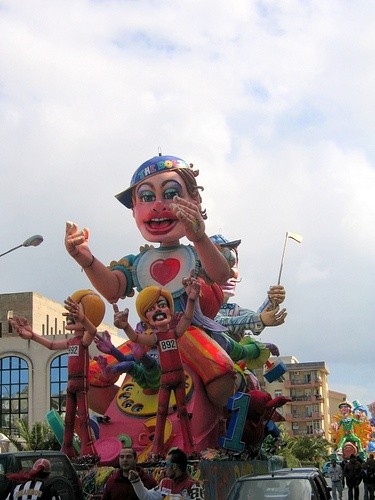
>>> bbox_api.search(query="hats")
[33,459,51,473]
[350,454,355,459]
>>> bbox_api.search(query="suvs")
[0,452,81,500]
[220,466,334,500]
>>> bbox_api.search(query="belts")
[332,480,340,482]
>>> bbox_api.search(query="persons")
[7,290,108,459]
[326,455,375,499]
[331,398,375,454]
[199,234,287,342]
[114,277,208,462]
[92,310,184,391]
[129,448,204,500]
[7,459,61,500]
[65,154,236,409]
[104,447,159,500]
